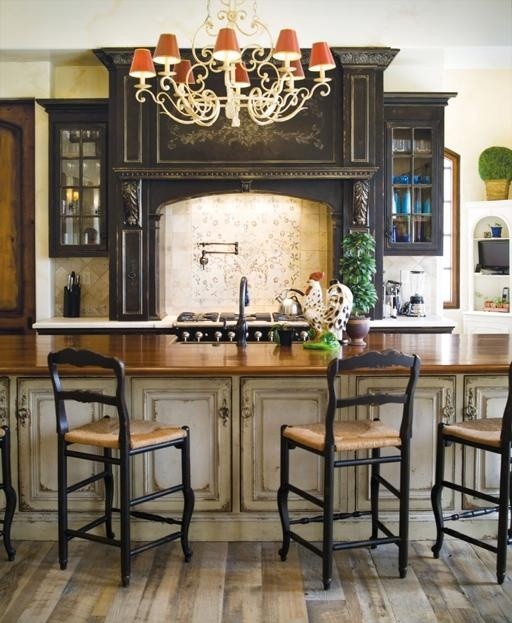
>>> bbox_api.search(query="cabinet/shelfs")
[48,117,111,257]
[384,117,443,257]
[1,374,125,515]
[354,370,512,514]
[129,372,353,523]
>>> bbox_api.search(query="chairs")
[47,347,194,585]
[276,350,421,589]
[431,363,512,585]
[0,424,17,560]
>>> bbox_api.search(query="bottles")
[393,190,430,243]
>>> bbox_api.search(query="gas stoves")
[175,310,311,345]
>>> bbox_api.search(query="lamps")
[129,1,340,128]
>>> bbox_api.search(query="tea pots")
[275,288,305,315]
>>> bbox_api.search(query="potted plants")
[339,232,378,346]
[479,147,512,201]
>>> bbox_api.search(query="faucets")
[235,276,249,346]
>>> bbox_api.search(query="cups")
[63,286,81,318]
[393,175,431,185]
[484,226,502,238]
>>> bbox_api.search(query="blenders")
[409,270,426,317]
